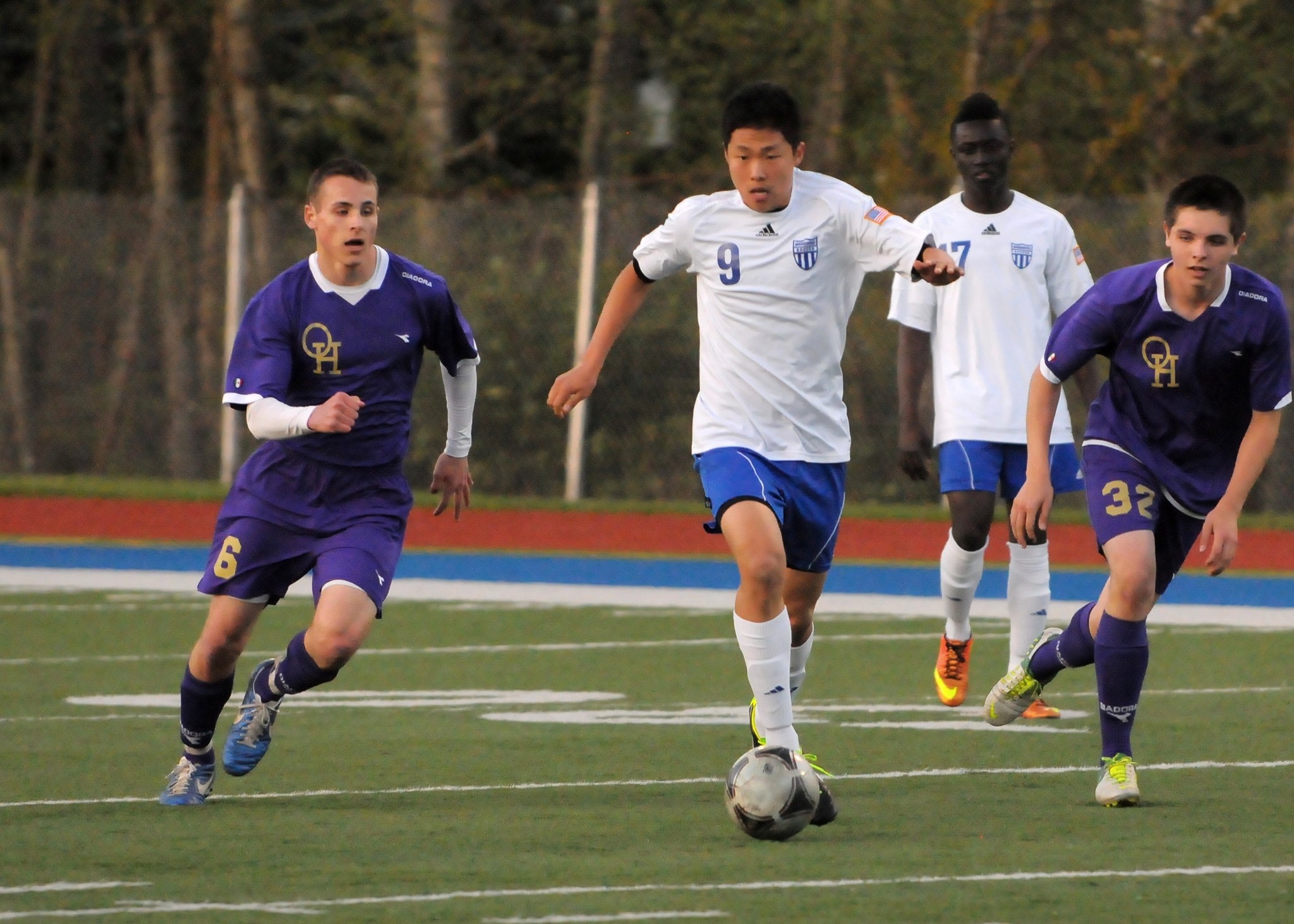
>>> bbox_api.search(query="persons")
[547,79,964,829]
[888,93,1105,718]
[161,157,482,806]
[983,175,1294,805]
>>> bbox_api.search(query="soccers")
[722,744,823,843]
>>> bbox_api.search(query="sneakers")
[934,632,973,706]
[223,657,286,777]
[160,756,216,805]
[1095,753,1141,806]
[983,628,1064,727]
[747,697,837,828]
[1021,700,1061,719]
